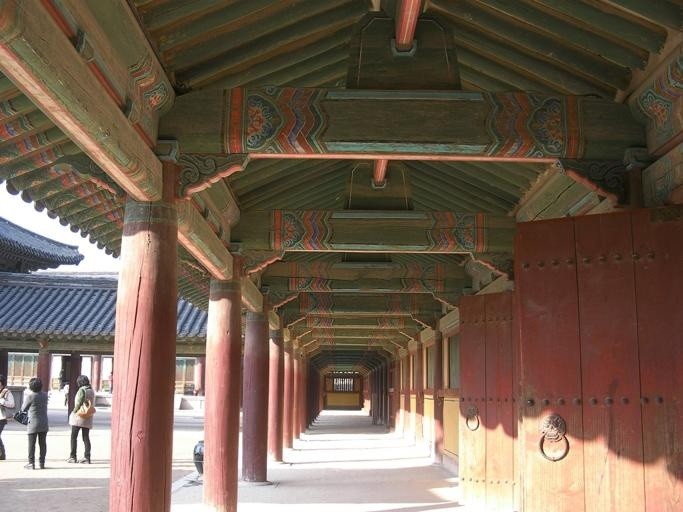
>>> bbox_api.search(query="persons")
[63,375,95,465]
[0,373,15,459]
[19,377,50,469]
[63,382,69,405]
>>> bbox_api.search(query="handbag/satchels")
[77,400,97,419]
[12,410,29,425]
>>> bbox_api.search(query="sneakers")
[64,456,91,465]
[23,463,46,471]
